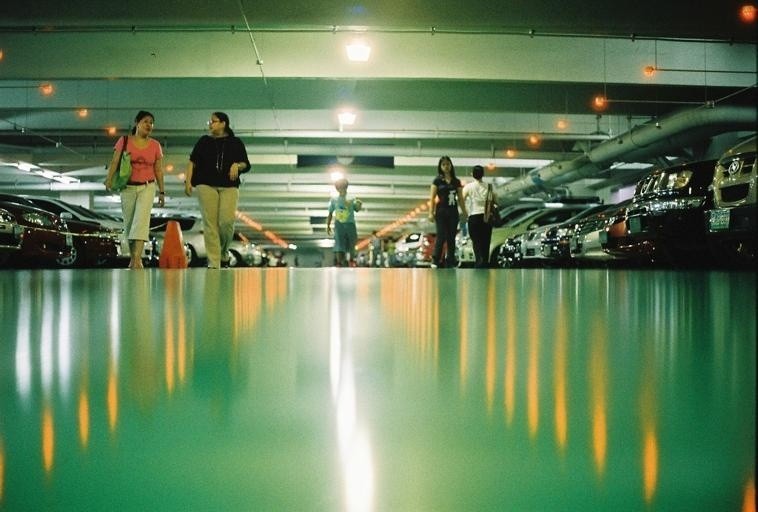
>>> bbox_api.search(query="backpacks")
[105,134,135,193]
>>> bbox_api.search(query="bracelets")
[159,191,165,194]
[237,162,240,169]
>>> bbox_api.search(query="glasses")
[206,118,220,125]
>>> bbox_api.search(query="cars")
[0,194,270,267]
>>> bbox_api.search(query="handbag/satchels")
[184,165,196,189]
[482,183,504,227]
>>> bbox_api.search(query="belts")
[126,178,155,186]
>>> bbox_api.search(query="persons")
[461,164,498,269]
[185,109,251,269]
[102,109,165,269]
[325,178,362,267]
[369,230,381,267]
[384,235,396,267]
[427,155,467,269]
